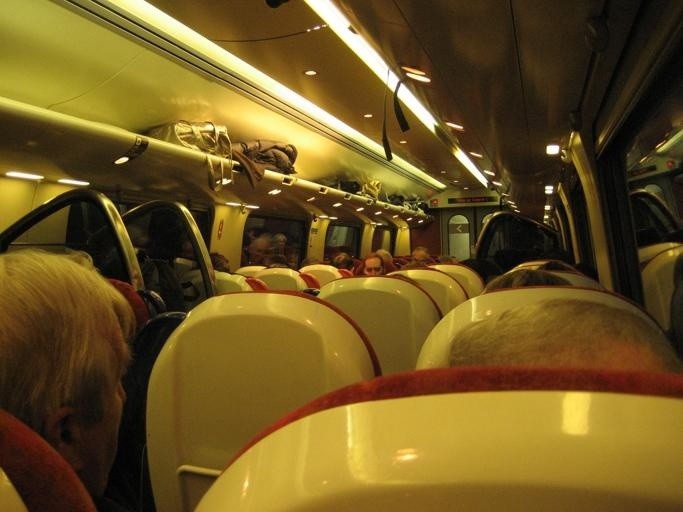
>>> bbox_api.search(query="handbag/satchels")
[144,120,230,158]
[231,139,296,174]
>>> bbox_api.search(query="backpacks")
[318,175,430,213]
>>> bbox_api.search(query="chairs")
[638,242,682,334]
[144,258,683,512]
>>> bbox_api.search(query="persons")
[70,222,354,281]
[201,244,578,296]
[58,249,95,272]
[446,298,682,377]
[0,242,136,512]
